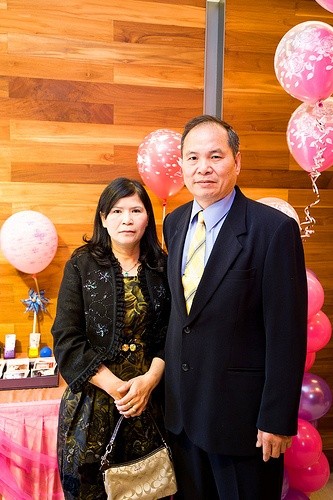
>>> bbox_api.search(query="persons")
[163,115,309,500]
[50,177,172,500]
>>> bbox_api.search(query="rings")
[131,407,137,413]
[127,402,132,409]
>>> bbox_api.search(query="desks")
[0,371,68,500]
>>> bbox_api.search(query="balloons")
[257,197,333,500]
[0,211,58,275]
[273,20,333,172]
[137,128,184,207]
[315,0,333,13]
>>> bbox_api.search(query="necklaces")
[122,263,138,276]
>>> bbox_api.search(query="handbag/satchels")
[99,404,178,500]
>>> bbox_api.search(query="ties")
[182,210,207,317]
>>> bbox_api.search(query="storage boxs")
[0,360,59,390]
